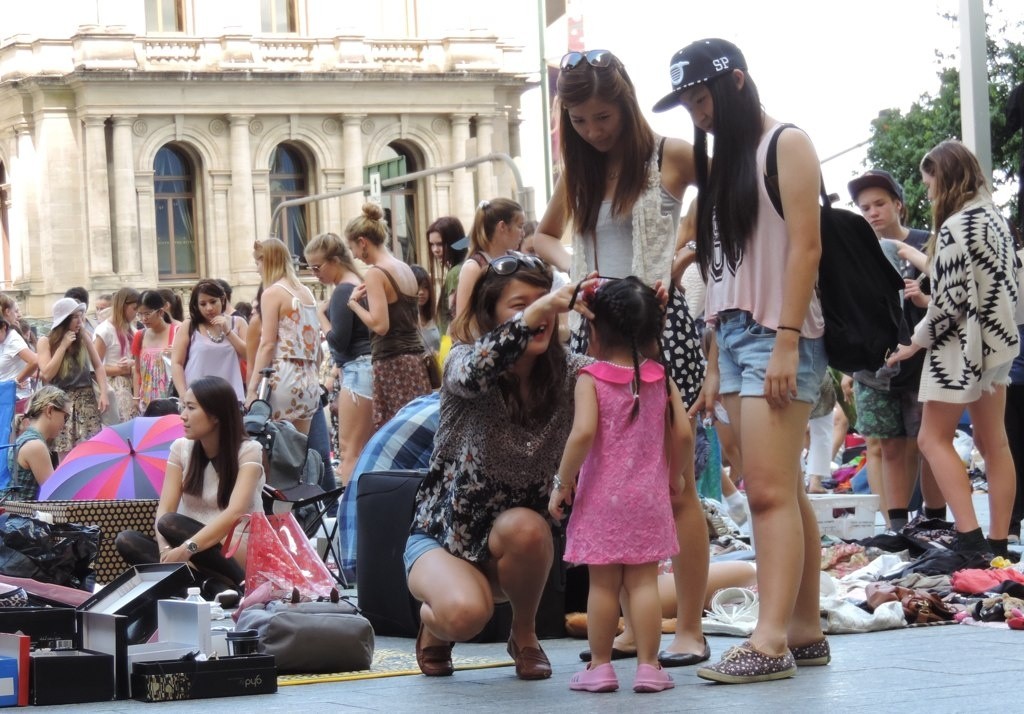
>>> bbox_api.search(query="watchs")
[185,539,199,554]
[554,478,573,491]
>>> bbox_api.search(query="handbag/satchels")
[868,582,960,625]
[219,513,342,624]
[0,513,101,593]
[92,377,120,426]
[231,598,373,674]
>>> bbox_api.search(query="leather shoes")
[416,623,456,675]
[506,633,551,677]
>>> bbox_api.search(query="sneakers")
[788,639,832,664]
[697,640,797,684]
[203,579,237,607]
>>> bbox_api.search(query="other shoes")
[580,644,637,662]
[633,665,677,692]
[808,484,829,494]
[569,663,618,691]
[657,636,711,666]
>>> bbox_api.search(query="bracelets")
[775,323,799,337]
[159,545,172,553]
[347,298,357,311]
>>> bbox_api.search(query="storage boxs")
[0,500,276,708]
[808,495,879,546]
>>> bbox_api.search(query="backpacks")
[763,125,913,371]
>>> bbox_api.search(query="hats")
[652,38,748,113]
[846,168,903,206]
[51,298,88,331]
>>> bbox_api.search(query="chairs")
[246,401,349,590]
[0,380,23,502]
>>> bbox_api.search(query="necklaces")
[203,325,226,343]
[604,171,620,180]
[419,320,427,327]
[601,357,649,378]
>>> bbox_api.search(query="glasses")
[50,405,72,423]
[560,50,628,82]
[306,257,332,274]
[253,240,263,252]
[481,253,550,286]
[136,309,160,319]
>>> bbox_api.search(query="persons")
[885,137,1021,557]
[652,38,829,683]
[548,275,696,693]
[244,239,321,484]
[115,376,270,605]
[303,230,373,488]
[404,252,599,681]
[533,50,710,665]
[0,201,1024,635]
[847,169,937,533]
